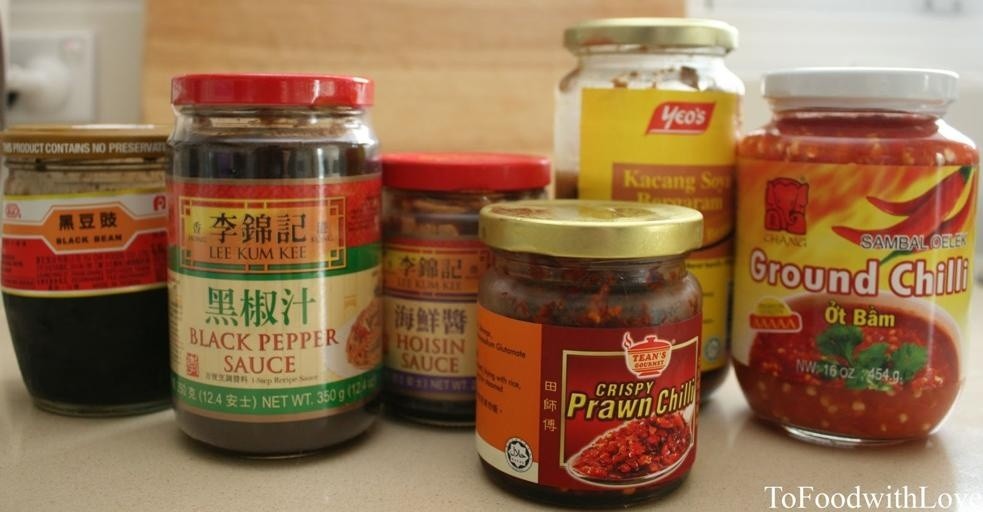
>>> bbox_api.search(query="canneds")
[378,151,554,427]
[476,199,698,510]
[552,18,744,402]
[733,65,978,448]
[164,75,387,460]
[0,121,172,417]
